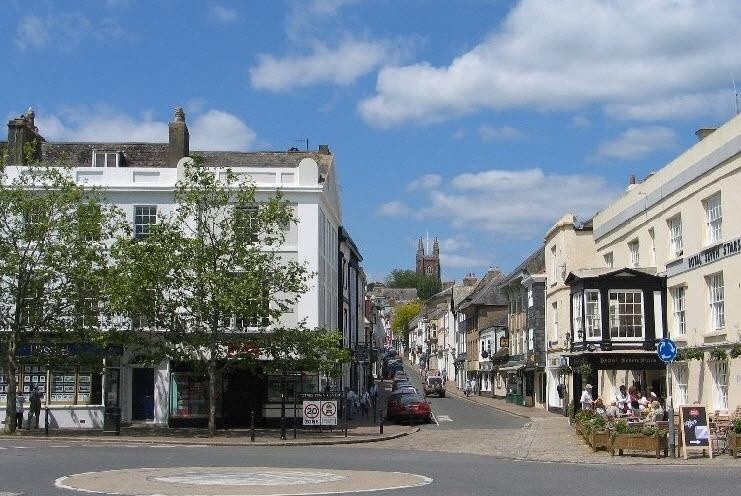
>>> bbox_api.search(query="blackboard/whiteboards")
[679,404,711,450]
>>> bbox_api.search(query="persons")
[579,383,670,425]
[343,378,386,421]
[24,385,44,430]
[461,377,480,397]
[419,356,447,385]
[15,389,27,430]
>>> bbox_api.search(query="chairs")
[597,407,741,455]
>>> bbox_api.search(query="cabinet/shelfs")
[0,363,94,406]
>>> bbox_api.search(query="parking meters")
[247,410,258,443]
[44,407,50,437]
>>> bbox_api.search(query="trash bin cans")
[506,393,523,405]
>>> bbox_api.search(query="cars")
[379,345,446,425]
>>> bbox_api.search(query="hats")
[586,384,593,388]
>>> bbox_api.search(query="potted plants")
[728,418,741,457]
[568,400,670,460]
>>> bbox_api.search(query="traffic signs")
[304,404,318,418]
